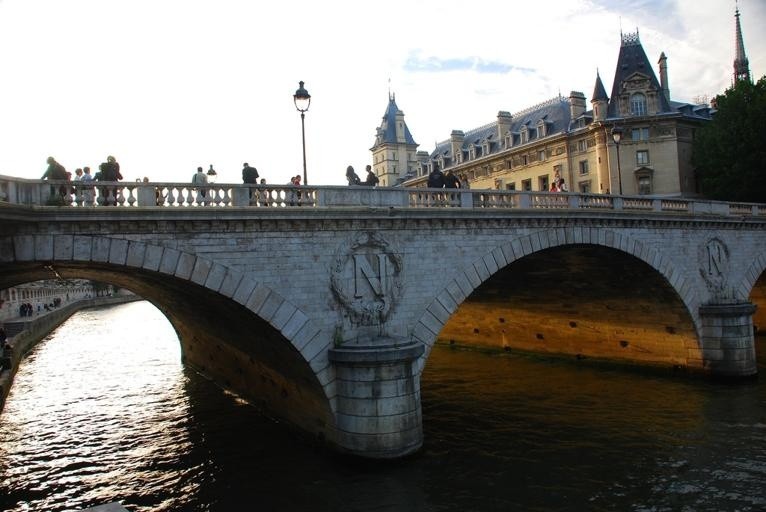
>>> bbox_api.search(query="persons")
[287,174,306,206]
[41,155,125,206]
[191,167,211,206]
[240,161,260,204]
[256,178,270,208]
[361,163,380,186]
[549,168,569,207]
[345,165,362,187]
[19,295,62,318]
[489,181,504,208]
[427,164,470,209]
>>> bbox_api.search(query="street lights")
[205,164,216,207]
[291,79,310,185]
[610,121,622,196]
